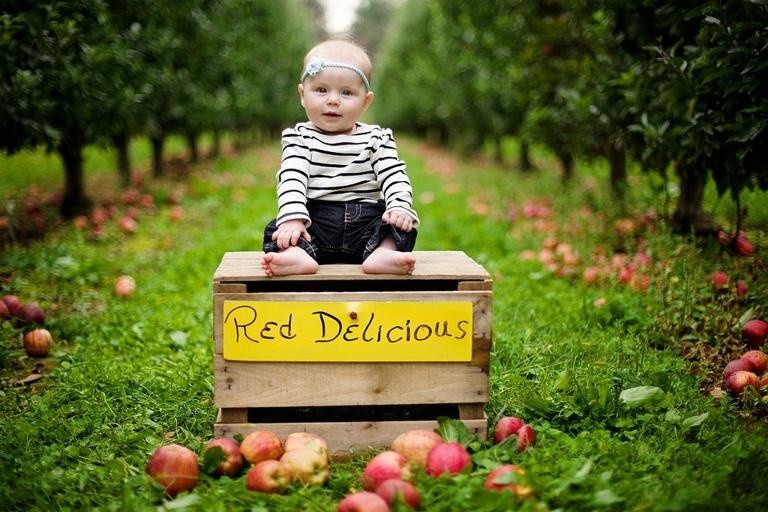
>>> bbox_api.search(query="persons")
[261,39,421,278]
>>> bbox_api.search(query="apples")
[0,147,767,512]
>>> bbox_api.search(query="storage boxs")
[213,249,493,452]
